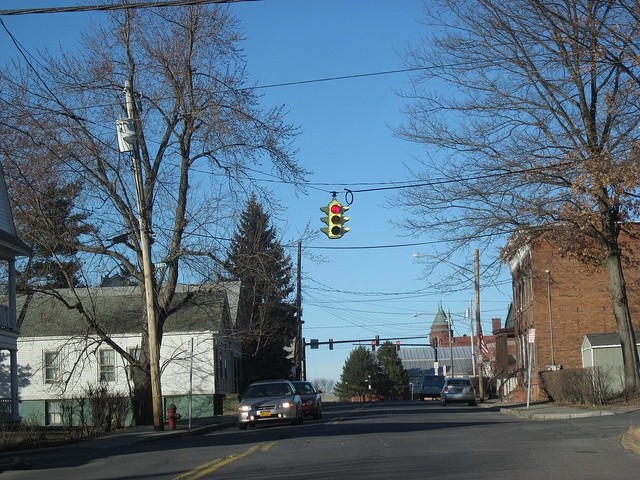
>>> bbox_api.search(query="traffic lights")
[372,340,376,351]
[329,338,333,350]
[320,198,350,239]
[396,340,400,351]
[376,335,379,346]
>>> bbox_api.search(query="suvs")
[420,376,445,401]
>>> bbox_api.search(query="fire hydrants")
[166,403,182,430]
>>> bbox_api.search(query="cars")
[440,378,476,406]
[291,381,322,419]
[238,380,302,430]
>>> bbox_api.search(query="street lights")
[413,309,453,378]
[412,249,489,402]
[546,270,562,372]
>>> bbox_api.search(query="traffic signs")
[187,336,197,351]
